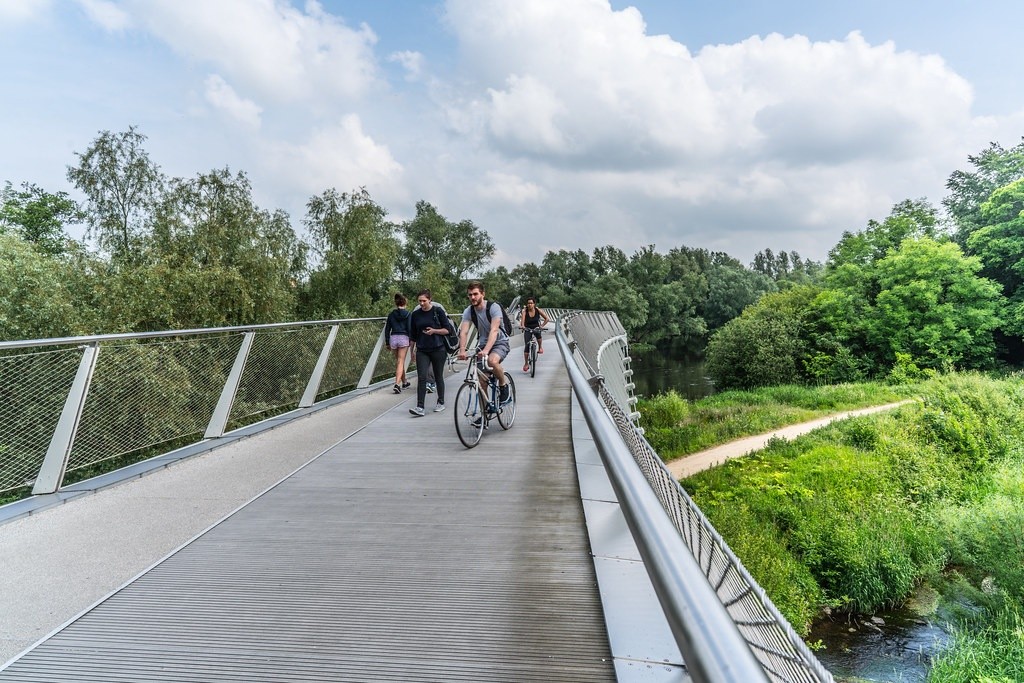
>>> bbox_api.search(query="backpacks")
[471,300,512,339]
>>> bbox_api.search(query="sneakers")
[409,407,425,415]
[425,382,436,392]
[499,383,511,404]
[401,382,412,389]
[471,416,489,430]
[433,402,445,412]
[393,384,401,393]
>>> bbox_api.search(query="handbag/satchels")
[433,307,461,353]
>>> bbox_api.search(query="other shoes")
[523,364,529,372]
[539,347,544,354]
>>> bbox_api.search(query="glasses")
[527,302,533,304]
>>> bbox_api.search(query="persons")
[457,282,511,426]
[385,288,451,415]
[519,298,549,371]
